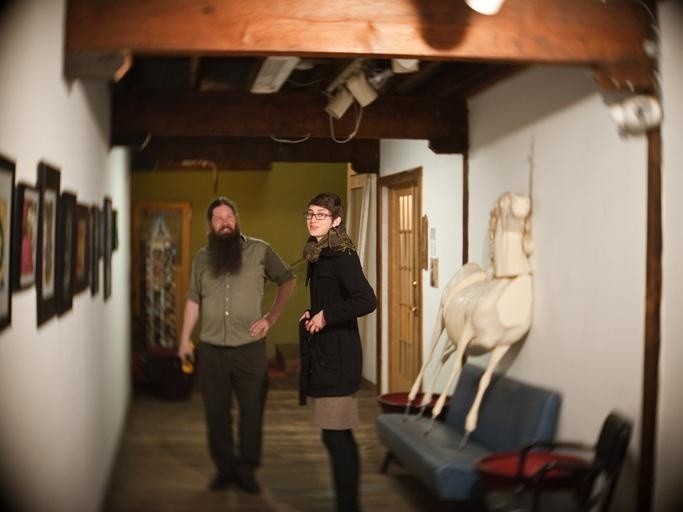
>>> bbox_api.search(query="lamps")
[323,58,418,120]
[466,0,505,15]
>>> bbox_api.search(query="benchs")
[375,363,560,511]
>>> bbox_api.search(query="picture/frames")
[0,156,116,332]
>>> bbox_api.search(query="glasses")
[303,211,334,220]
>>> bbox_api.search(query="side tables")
[471,452,589,512]
[376,393,452,419]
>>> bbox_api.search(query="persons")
[177,196,296,497]
[295,192,377,512]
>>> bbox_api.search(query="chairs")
[515,411,631,511]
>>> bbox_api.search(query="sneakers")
[235,473,258,492]
[211,471,232,487]
